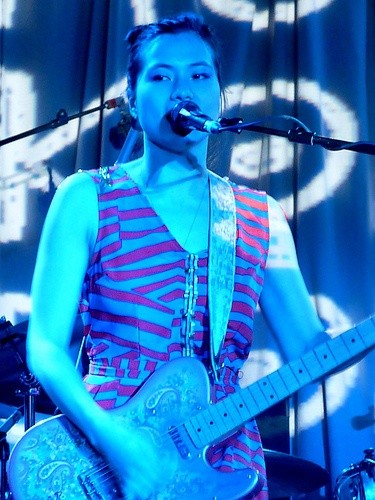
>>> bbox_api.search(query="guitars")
[6,312,375,500]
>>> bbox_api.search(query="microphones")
[171,100,223,135]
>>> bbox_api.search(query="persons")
[24,13,375,499]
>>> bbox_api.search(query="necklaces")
[183,184,207,249]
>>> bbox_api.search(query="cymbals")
[262,449,330,496]
[0,317,57,415]
